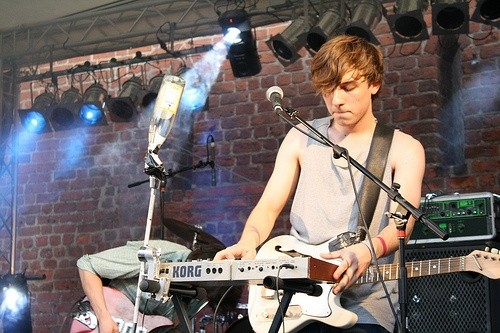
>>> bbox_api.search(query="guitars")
[247,232,500,333]
[68,285,175,333]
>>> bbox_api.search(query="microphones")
[211,138,216,187]
[265,86,284,114]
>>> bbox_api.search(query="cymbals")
[162,218,226,252]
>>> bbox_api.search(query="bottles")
[147,74,186,151]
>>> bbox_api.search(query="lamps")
[264,12,318,68]
[104,75,144,124]
[140,69,166,109]
[384,0,431,44]
[75,81,108,128]
[335,3,384,47]
[176,65,210,111]
[468,0,500,31]
[296,6,347,59]
[48,85,81,133]
[430,0,470,36]
[16,81,58,136]
[0,272,28,314]
[217,8,264,78]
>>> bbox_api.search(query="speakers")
[403,242,500,333]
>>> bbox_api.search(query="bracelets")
[362,240,376,263]
[376,237,387,257]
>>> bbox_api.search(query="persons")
[212,35,425,333]
[77,240,242,333]
